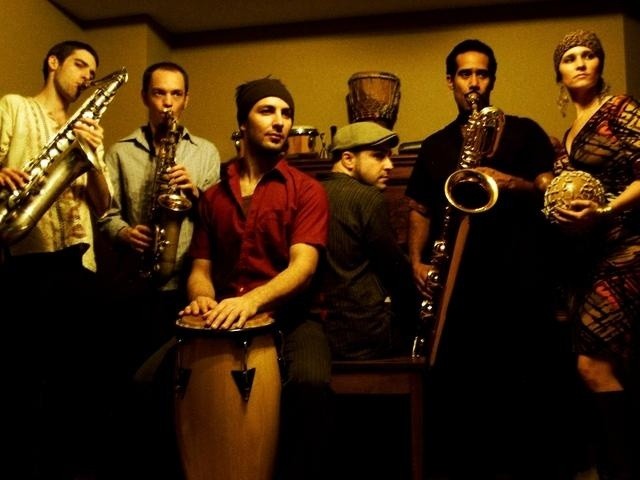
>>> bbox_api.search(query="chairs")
[328,213,470,479]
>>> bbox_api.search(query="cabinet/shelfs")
[283,153,417,201]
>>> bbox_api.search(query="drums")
[175,310,281,480]
[233,124,325,159]
[347,71,401,122]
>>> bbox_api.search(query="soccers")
[543,169,606,228]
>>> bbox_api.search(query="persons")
[315,117,410,366]
[1,40,115,479]
[401,38,555,479]
[534,29,640,480]
[126,74,330,424]
[101,62,219,480]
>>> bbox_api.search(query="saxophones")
[412,94,505,367]
[0,65,128,245]
[138,108,192,286]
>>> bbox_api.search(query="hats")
[234,79,295,127]
[329,121,400,154]
[553,27,606,81]
